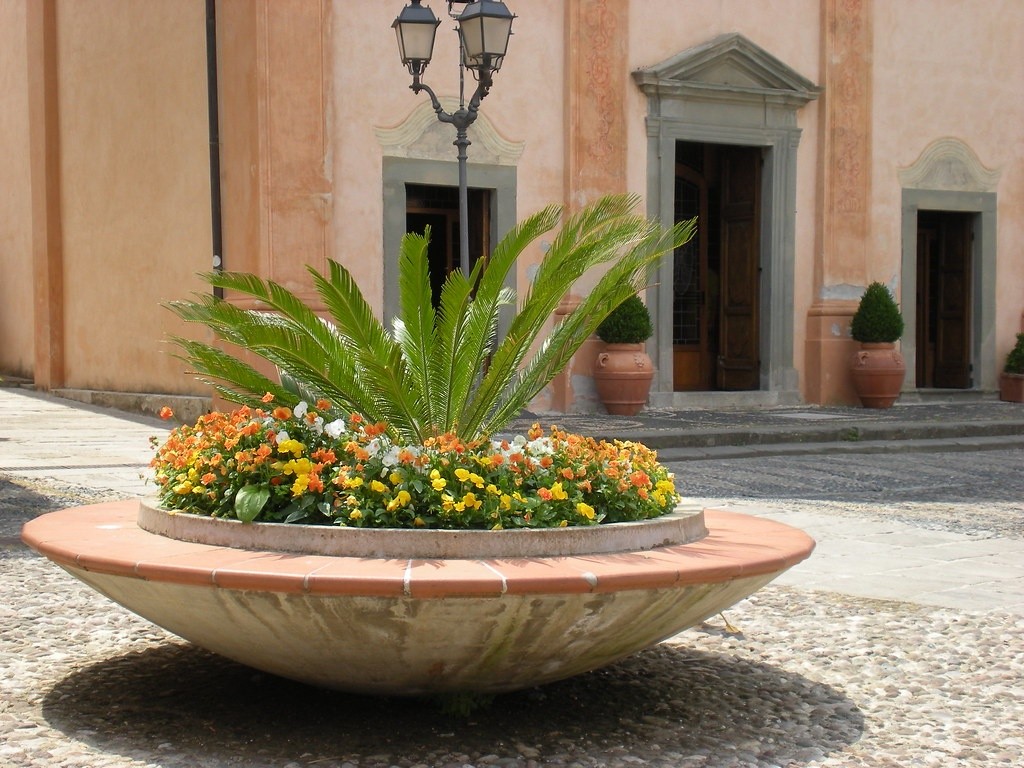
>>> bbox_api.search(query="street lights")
[390,0,521,307]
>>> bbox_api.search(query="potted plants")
[848,279,906,409]
[999,331,1024,403]
[594,284,654,416]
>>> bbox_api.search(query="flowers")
[144,193,679,526]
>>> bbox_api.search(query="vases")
[24,492,814,695]
